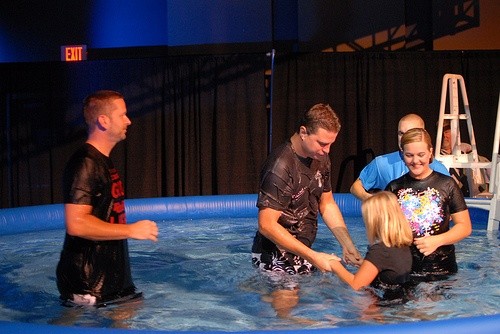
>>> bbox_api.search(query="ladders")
[434,73,500,240]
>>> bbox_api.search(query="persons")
[251,104,473,322]
[56,89,159,304]
